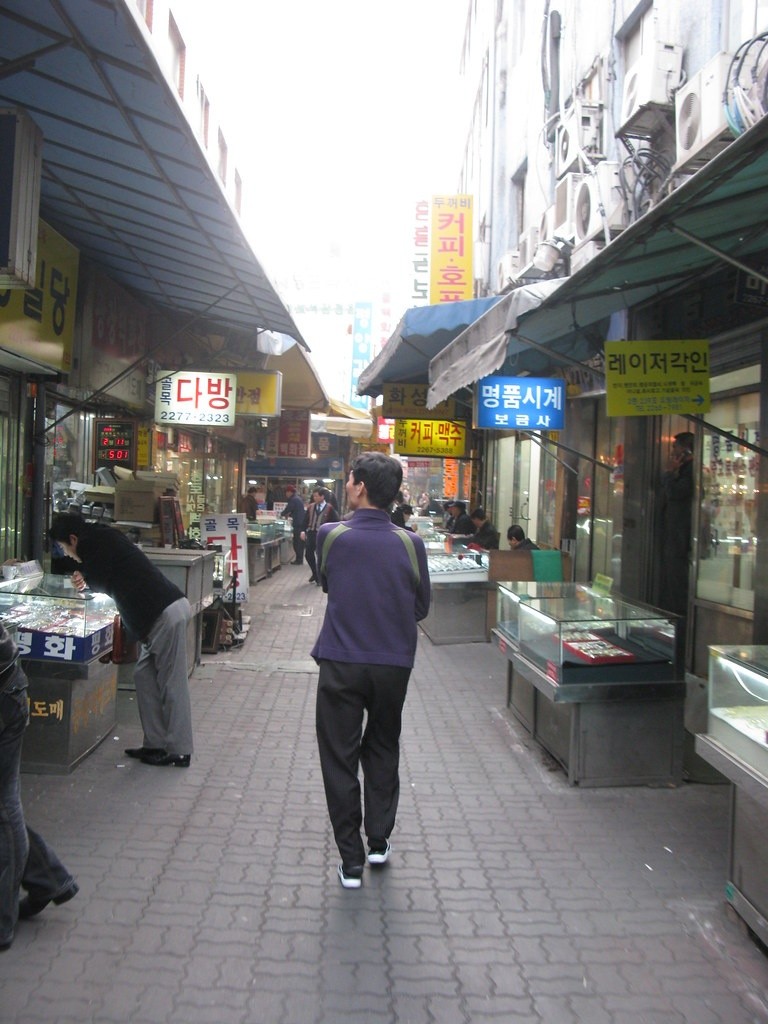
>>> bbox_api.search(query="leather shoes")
[18,881,79,916]
[124,747,163,758]
[139,751,190,768]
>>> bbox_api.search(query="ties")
[317,504,322,513]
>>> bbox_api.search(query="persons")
[0,618,79,952]
[283,480,340,587]
[241,487,259,521]
[54,511,195,767]
[316,451,432,890]
[440,501,540,550]
[393,485,417,533]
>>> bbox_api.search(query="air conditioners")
[673,50,746,174]
[516,228,544,279]
[621,42,681,137]
[574,159,634,246]
[497,251,524,295]
[554,173,590,237]
[555,99,603,180]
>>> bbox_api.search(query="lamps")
[533,235,573,271]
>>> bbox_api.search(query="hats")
[449,502,466,509]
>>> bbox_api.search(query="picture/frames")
[173,499,184,547]
[159,495,177,549]
[202,609,223,654]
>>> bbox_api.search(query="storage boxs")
[113,479,166,522]
[113,465,135,480]
[696,553,756,614]
[136,470,181,490]
[84,485,114,504]
[96,467,116,487]
[141,524,161,538]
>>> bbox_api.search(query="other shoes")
[309,575,320,584]
[291,559,303,565]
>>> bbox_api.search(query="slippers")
[337,862,363,886]
[366,839,392,863]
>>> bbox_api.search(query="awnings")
[2,4,330,438]
[310,414,373,440]
[330,277,606,420]
[513,116,768,338]
